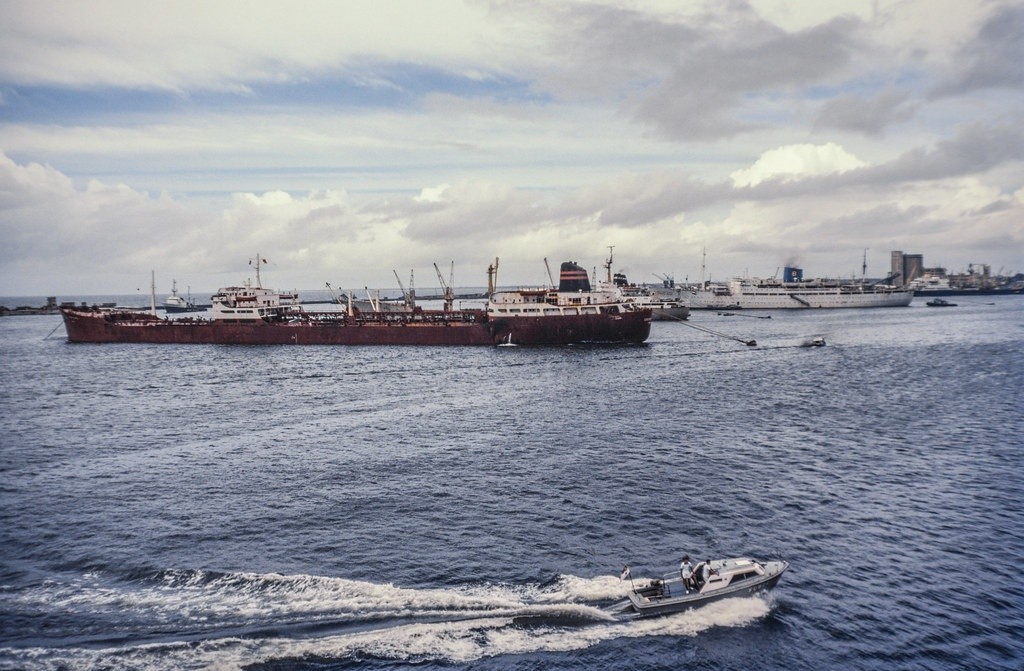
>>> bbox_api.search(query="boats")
[654,272,914,308]
[161,278,207,313]
[61,260,648,351]
[927,298,958,307]
[207,256,304,321]
[589,272,690,321]
[626,557,791,616]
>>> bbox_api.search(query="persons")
[680,555,701,594]
[703,559,716,583]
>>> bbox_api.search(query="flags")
[619,566,630,583]
